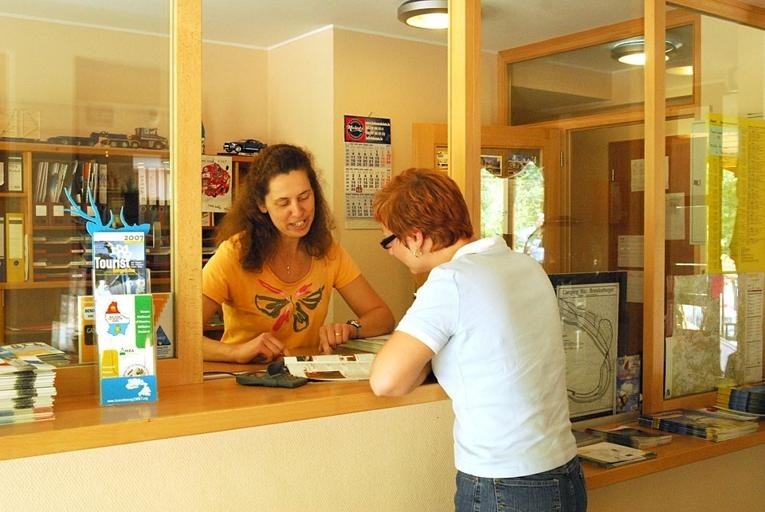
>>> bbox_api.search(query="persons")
[369,168,587,512]
[202,144,395,364]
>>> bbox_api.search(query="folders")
[123,168,172,227]
[1,212,28,284]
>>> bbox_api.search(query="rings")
[335,333,341,335]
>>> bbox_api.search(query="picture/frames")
[545,269,629,423]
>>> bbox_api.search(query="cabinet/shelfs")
[0,141,259,344]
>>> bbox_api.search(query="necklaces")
[278,251,290,270]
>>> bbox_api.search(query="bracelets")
[346,320,362,339]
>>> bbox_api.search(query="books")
[283,353,375,384]
[335,333,391,354]
[572,355,765,470]
[1,136,217,425]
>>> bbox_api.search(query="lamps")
[665,59,695,77]
[609,32,685,68]
[395,1,450,33]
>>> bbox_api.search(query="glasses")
[379,233,394,250]
